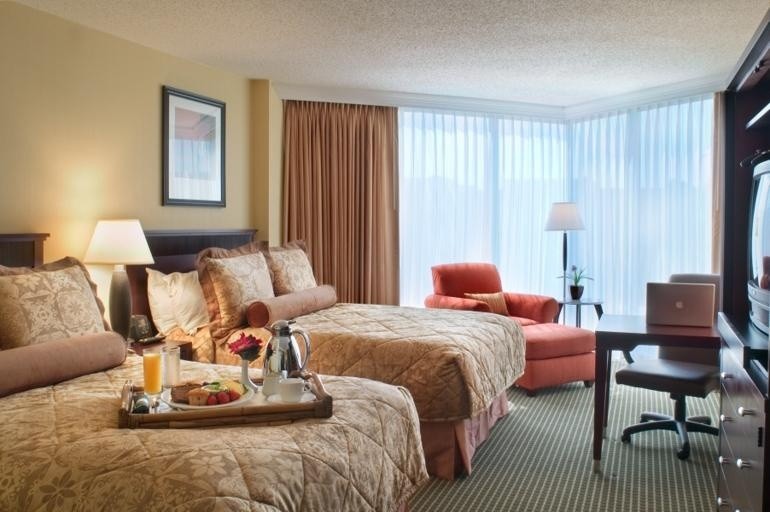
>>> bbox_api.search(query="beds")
[122,229,529,479]
[1,227,431,510]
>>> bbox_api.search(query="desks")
[552,298,605,327]
[589,309,721,472]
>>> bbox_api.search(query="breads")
[172,380,203,403]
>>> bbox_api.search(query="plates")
[161,387,254,408]
[265,393,317,403]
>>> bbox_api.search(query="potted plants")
[557,262,591,300]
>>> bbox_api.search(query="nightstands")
[126,337,194,361]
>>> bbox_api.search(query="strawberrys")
[228,390,241,401]
[207,395,217,405]
[216,391,230,405]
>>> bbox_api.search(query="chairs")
[613,271,725,459]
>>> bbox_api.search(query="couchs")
[424,259,595,396]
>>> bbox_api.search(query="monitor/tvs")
[746,160,770,336]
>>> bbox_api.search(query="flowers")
[225,329,263,362]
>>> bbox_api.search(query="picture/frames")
[160,84,227,207]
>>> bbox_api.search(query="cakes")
[186,387,210,405]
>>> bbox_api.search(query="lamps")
[546,200,584,326]
[82,219,154,340]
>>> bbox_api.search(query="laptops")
[647,283,715,328]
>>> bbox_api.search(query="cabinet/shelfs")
[703,343,770,511]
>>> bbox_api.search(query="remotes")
[137,336,166,345]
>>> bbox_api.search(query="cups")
[276,376,312,404]
[143,348,163,399]
[162,345,181,390]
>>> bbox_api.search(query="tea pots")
[259,319,311,392]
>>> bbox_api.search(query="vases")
[241,359,258,391]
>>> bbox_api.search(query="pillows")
[0,255,112,351]
[262,239,319,296]
[194,236,276,347]
[245,282,337,327]
[464,289,511,315]
[164,266,210,336]
[144,265,177,336]
[0,327,127,400]
